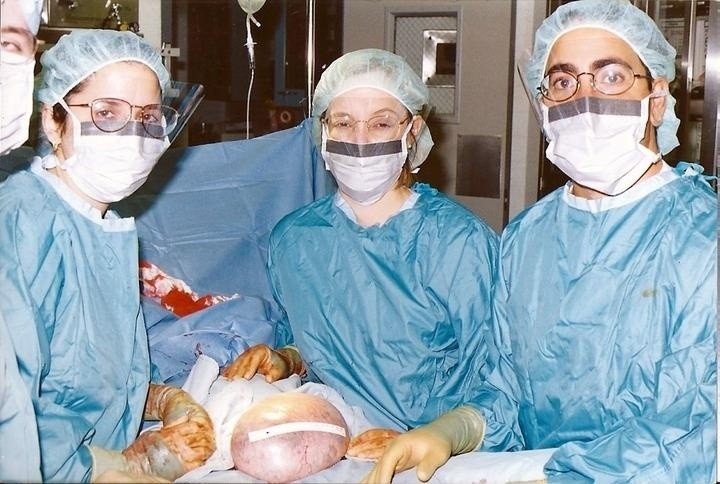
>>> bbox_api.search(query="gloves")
[144,385,215,435]
[221,342,306,384]
[358,408,487,484]
[86,413,215,481]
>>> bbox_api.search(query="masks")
[535,89,668,195]
[1,42,42,154]
[44,89,171,203]
[319,116,419,206]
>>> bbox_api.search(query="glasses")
[320,114,410,141]
[0,26,46,66]
[538,63,653,102]
[67,98,180,139]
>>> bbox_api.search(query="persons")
[1,26,221,481]
[357,0,720,483]
[221,45,501,463]
[0,1,48,481]
